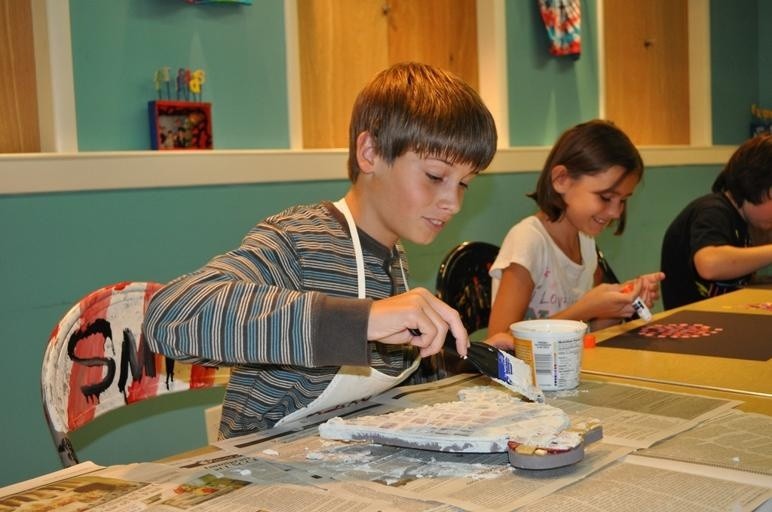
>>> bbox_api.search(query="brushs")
[408,328,536,402]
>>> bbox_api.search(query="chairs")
[427,239,508,381]
[39,281,231,469]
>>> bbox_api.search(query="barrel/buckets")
[510,317,587,389]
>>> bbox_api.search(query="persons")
[659,133,771,313]
[141,57,499,438]
[484,116,667,343]
